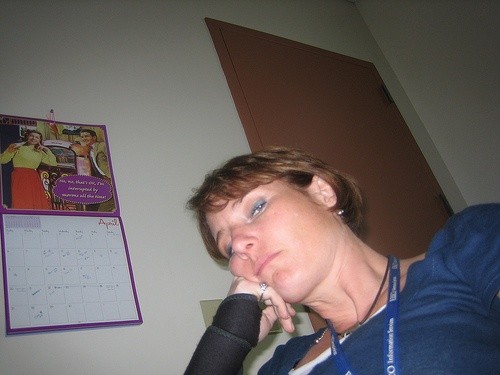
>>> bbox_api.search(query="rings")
[259,281,268,302]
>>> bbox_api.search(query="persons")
[67,129,110,210]
[1,130,58,210]
[182,145,500,374]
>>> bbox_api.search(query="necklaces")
[323,255,391,340]
